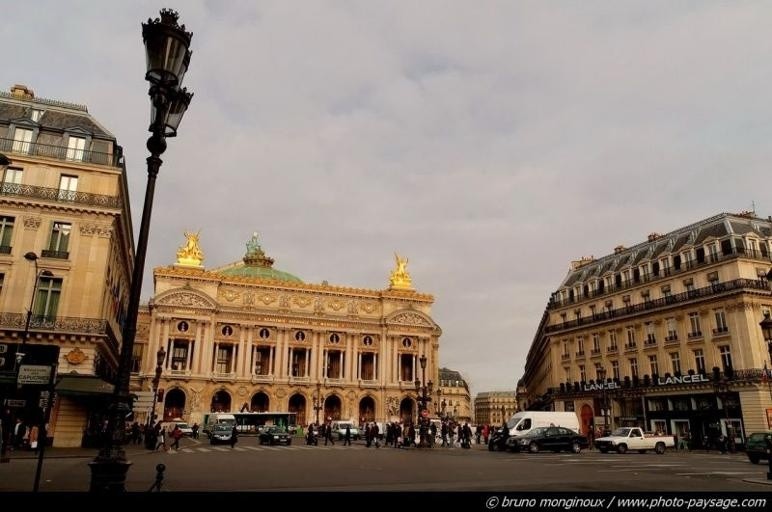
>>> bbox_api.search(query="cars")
[210,424,236,445]
[506,427,590,454]
[307,424,323,439]
[259,426,292,446]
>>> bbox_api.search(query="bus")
[203,412,298,436]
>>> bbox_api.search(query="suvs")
[745,431,772,463]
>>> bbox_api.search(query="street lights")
[415,355,434,448]
[433,388,447,416]
[145,345,166,451]
[760,310,772,366]
[312,382,325,424]
[595,366,614,436]
[85,7,194,511]
[713,378,738,455]
[0,251,40,464]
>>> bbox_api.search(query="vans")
[206,414,237,438]
[167,422,192,437]
[497,411,581,447]
[329,421,358,441]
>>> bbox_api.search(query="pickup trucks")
[594,427,677,456]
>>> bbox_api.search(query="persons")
[21,423,30,445]
[14,417,25,449]
[687,431,694,452]
[127,420,162,451]
[231,424,239,448]
[586,425,594,450]
[323,422,335,446]
[169,424,183,452]
[29,424,39,446]
[344,425,352,447]
[156,428,167,452]
[363,421,509,450]
[190,422,199,439]
[307,423,314,445]
[719,433,728,454]
